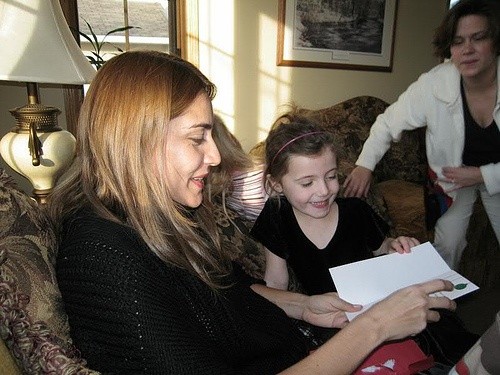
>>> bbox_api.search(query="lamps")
[0,0,99,205]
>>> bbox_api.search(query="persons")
[343,0,500,331]
[51,51,457,375]
[250,117,431,353]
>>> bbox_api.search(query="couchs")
[0,96,490,375]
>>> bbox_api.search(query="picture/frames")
[275,0,399,74]
